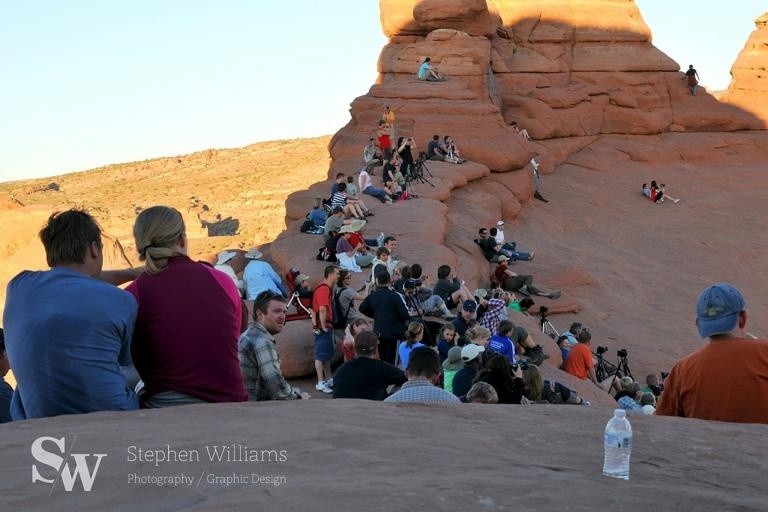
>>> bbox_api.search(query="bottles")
[604,409,631,477]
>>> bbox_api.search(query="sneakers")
[356,210,375,222]
[314,377,333,395]
[378,231,385,247]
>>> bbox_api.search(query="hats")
[349,219,366,232]
[461,344,486,363]
[496,220,505,227]
[442,345,464,371]
[461,299,477,314]
[497,255,510,263]
[244,248,263,261]
[215,251,238,265]
[294,274,310,285]
[338,225,355,234]
[695,282,746,339]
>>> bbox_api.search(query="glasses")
[258,288,287,306]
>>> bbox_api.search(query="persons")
[0,328,13,424]
[242,247,285,302]
[648,281,767,426]
[123,206,246,410]
[680,64,700,96]
[416,56,444,84]
[239,289,309,401]
[3,206,139,424]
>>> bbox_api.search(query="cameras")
[596,346,608,354]
[408,139,412,141]
[405,277,423,288]
[617,349,628,356]
[540,307,548,312]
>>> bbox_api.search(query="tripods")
[540,319,560,336]
[403,158,435,198]
[607,359,635,395]
[596,359,608,377]
